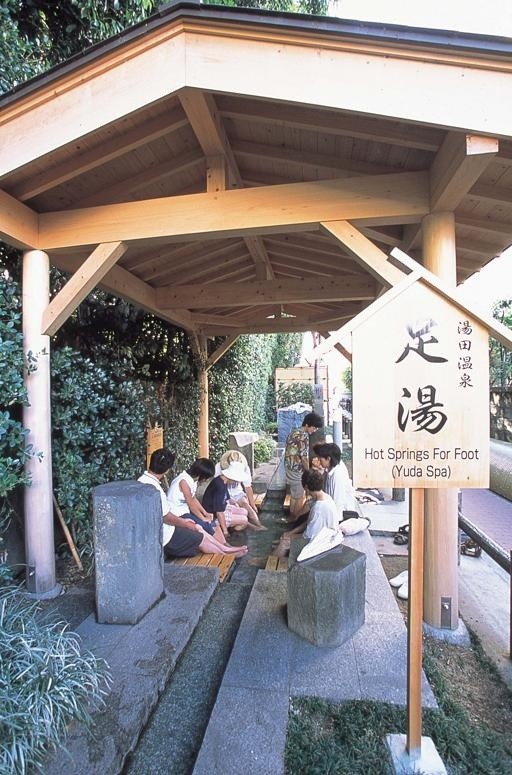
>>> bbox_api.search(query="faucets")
[393,523,411,545]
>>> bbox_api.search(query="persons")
[138,413,357,566]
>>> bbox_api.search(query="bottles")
[220,460,250,483]
[219,450,249,471]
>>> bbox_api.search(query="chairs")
[389,568,408,588]
[395,580,408,600]
